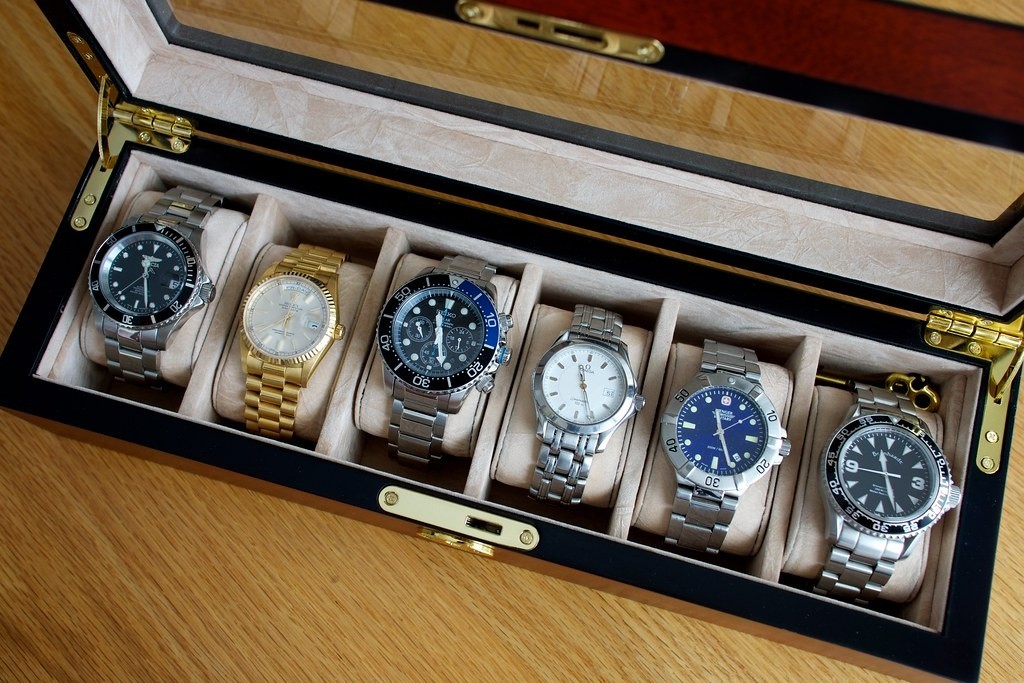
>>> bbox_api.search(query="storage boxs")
[0,0,1024,683]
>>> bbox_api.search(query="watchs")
[812,379,963,614]
[88,182,230,394]
[527,300,646,506]
[374,252,512,470]
[236,242,349,440]
[657,340,792,558]
